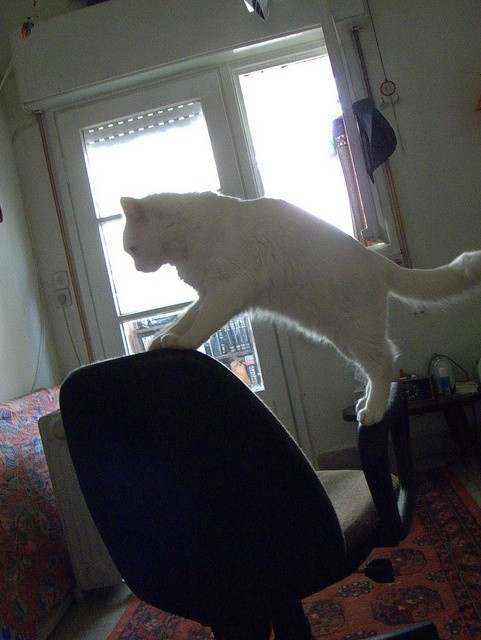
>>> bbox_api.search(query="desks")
[340,378,451,474]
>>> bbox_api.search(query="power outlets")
[52,287,72,309]
[49,271,67,288]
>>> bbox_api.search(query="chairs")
[57,347,441,640]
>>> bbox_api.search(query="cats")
[120,191,481,426]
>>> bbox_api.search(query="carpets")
[86,462,481,640]
